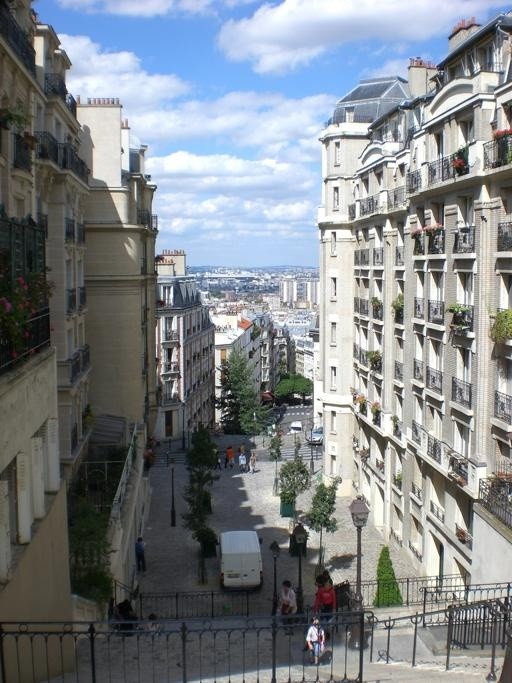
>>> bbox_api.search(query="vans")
[286,420,303,434]
[221,529,266,590]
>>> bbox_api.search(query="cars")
[306,428,324,446]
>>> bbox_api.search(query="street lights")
[178,402,188,452]
[348,494,371,649]
[167,458,178,528]
[270,540,282,615]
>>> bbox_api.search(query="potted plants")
[279,492,296,516]
[448,304,471,326]
[370,296,381,310]
[393,293,404,319]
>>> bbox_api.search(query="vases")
[415,234,420,239]
[426,230,441,236]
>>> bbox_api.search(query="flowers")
[411,227,422,238]
[423,223,443,236]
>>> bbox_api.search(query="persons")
[280,580,297,635]
[135,535,147,573]
[145,614,162,636]
[314,579,336,641]
[213,444,257,474]
[315,570,332,586]
[308,617,325,666]
